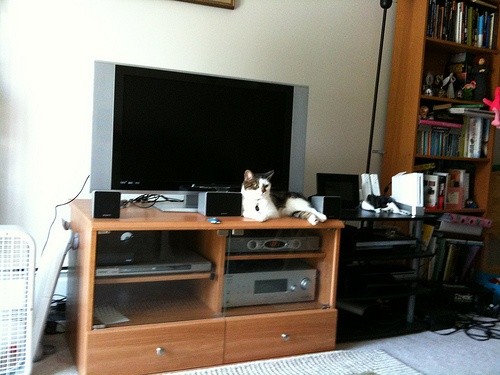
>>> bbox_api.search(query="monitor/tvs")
[89,62,309,212]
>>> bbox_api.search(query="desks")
[331,210,437,340]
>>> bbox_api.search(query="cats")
[241,169,329,227]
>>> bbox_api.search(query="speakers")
[312,196,342,217]
[91,190,122,218]
[196,192,242,217]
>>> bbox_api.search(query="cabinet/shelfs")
[65,198,345,375]
[379,0,500,286]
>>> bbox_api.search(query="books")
[424,213,492,284]
[448,52,490,100]
[416,103,495,157]
[426,0,497,49]
[425,167,469,212]
